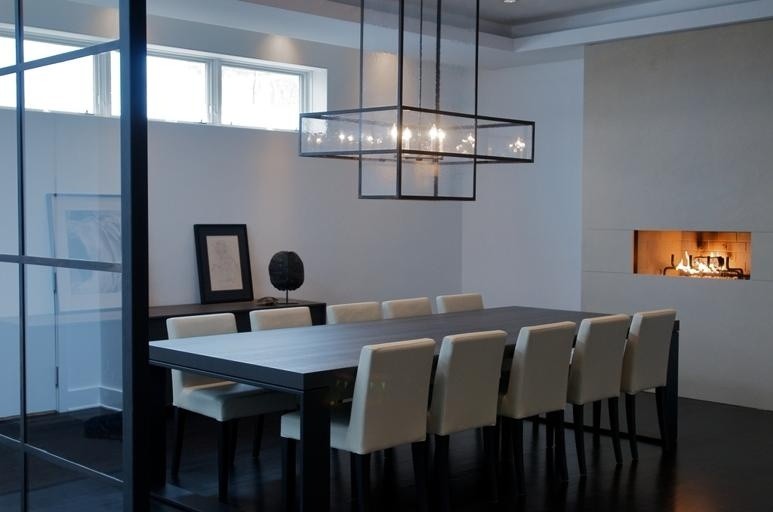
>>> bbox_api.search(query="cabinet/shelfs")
[0,297,327,442]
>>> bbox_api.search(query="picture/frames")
[45,193,123,314]
[193,223,254,305]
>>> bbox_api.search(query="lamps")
[298,0,535,201]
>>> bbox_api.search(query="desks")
[149,306,617,512]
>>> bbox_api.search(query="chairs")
[566,313,631,474]
[622,309,678,469]
[497,321,576,511]
[248,307,312,332]
[379,297,432,319]
[326,302,381,325]
[428,329,507,511]
[435,294,484,313]
[281,337,435,511]
[165,313,271,501]
